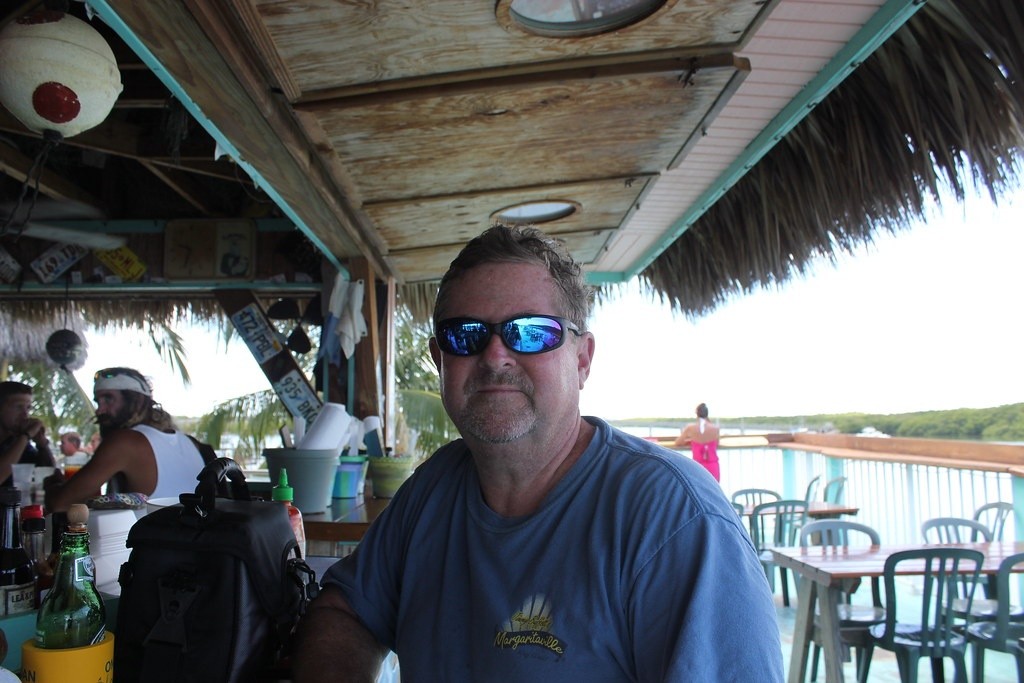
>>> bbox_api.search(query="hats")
[93,369,151,396]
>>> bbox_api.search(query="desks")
[770,542,1024,683]
[293,487,391,541]
[734,501,859,606]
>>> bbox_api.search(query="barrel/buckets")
[370,457,416,498]
[260,447,370,514]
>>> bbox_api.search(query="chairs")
[730,475,1024,683]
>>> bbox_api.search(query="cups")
[34,467,55,496]
[63,464,84,480]
[10,464,36,496]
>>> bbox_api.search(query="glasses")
[434,313,582,357]
[94,369,146,392]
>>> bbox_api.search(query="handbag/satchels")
[109,456,318,683]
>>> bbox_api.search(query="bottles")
[0,487,107,650]
[271,467,306,561]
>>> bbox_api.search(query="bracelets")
[18,431,30,441]
[36,439,49,450]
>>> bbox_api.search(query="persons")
[273,224,784,683]
[0,381,56,489]
[674,403,720,482]
[43,367,205,515]
[56,431,101,467]
